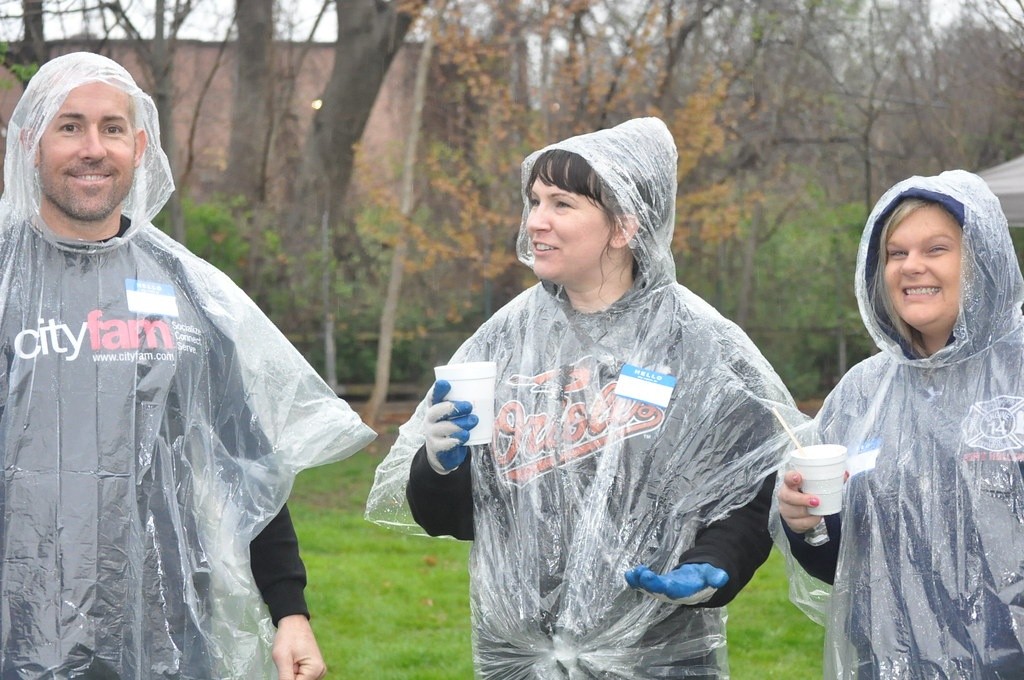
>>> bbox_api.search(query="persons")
[405,133,781,680]
[0,50,327,680]
[777,185,1024,680]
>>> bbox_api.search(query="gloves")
[624,563,729,605]
[425,379,479,475]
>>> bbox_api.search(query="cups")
[434,361,496,447]
[788,444,846,518]
